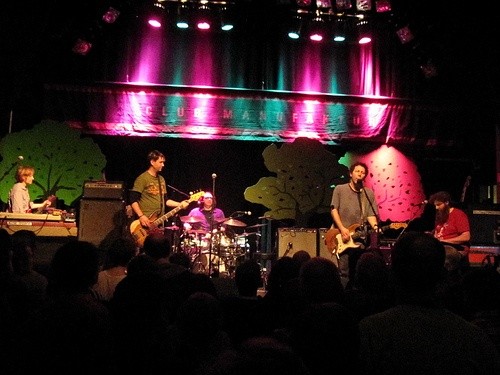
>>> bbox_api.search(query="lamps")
[195,6,212,30]
[220,7,234,31]
[147,7,162,28]
[356,14,372,45]
[288,15,304,40]
[307,17,324,43]
[176,9,189,29]
[333,18,346,42]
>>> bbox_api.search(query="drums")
[182,231,245,278]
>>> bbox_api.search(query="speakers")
[276,228,338,270]
[77,198,127,248]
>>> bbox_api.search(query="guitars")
[324,222,409,257]
[26,194,57,213]
[125,189,205,249]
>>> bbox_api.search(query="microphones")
[237,211,251,215]
[212,173,217,178]
[289,243,292,249]
[357,179,363,192]
[14,156,23,165]
[415,200,429,206]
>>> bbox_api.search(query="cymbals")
[188,228,206,234]
[180,215,204,223]
[164,226,182,231]
[248,222,268,229]
[237,232,257,237]
[220,218,249,226]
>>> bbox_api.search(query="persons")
[129,149,189,255]
[429,191,471,244]
[7,165,51,214]
[0,229,500,375]
[188,191,226,272]
[331,163,381,287]
